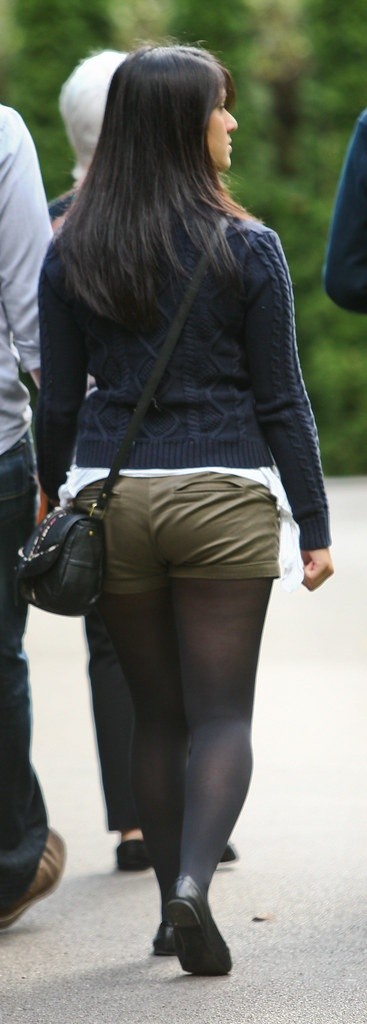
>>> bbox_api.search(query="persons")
[324,109,367,314]
[31,44,338,975]
[0,98,66,928]
[43,49,245,869]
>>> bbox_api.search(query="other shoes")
[154,916,179,958]
[117,839,151,872]
[0,825,66,932]
[217,842,235,864]
[165,872,231,975]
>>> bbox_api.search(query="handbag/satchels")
[15,507,105,617]
